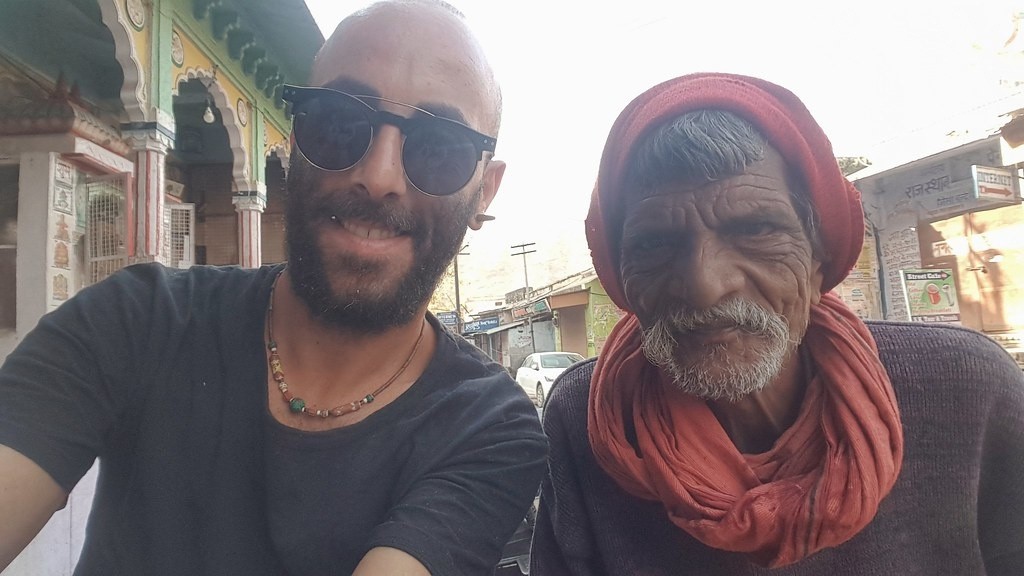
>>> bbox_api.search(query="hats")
[585,71,865,316]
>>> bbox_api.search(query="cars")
[516,352,587,408]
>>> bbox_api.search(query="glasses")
[281,84,497,198]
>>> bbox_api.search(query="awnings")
[461,315,552,338]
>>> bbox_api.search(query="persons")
[0,0,549,576]
[529,72,1024,576]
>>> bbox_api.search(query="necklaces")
[268,271,425,418]
